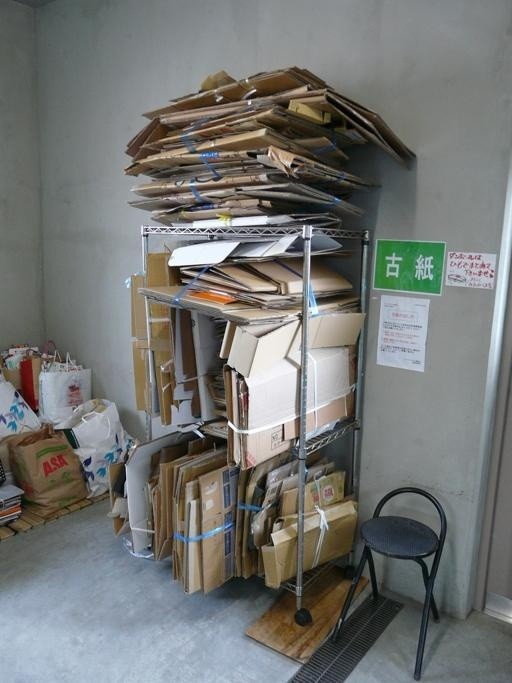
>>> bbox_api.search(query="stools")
[332,483,448,681]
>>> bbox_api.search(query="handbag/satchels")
[11,337,130,509]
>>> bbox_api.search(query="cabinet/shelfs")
[138,221,371,611]
